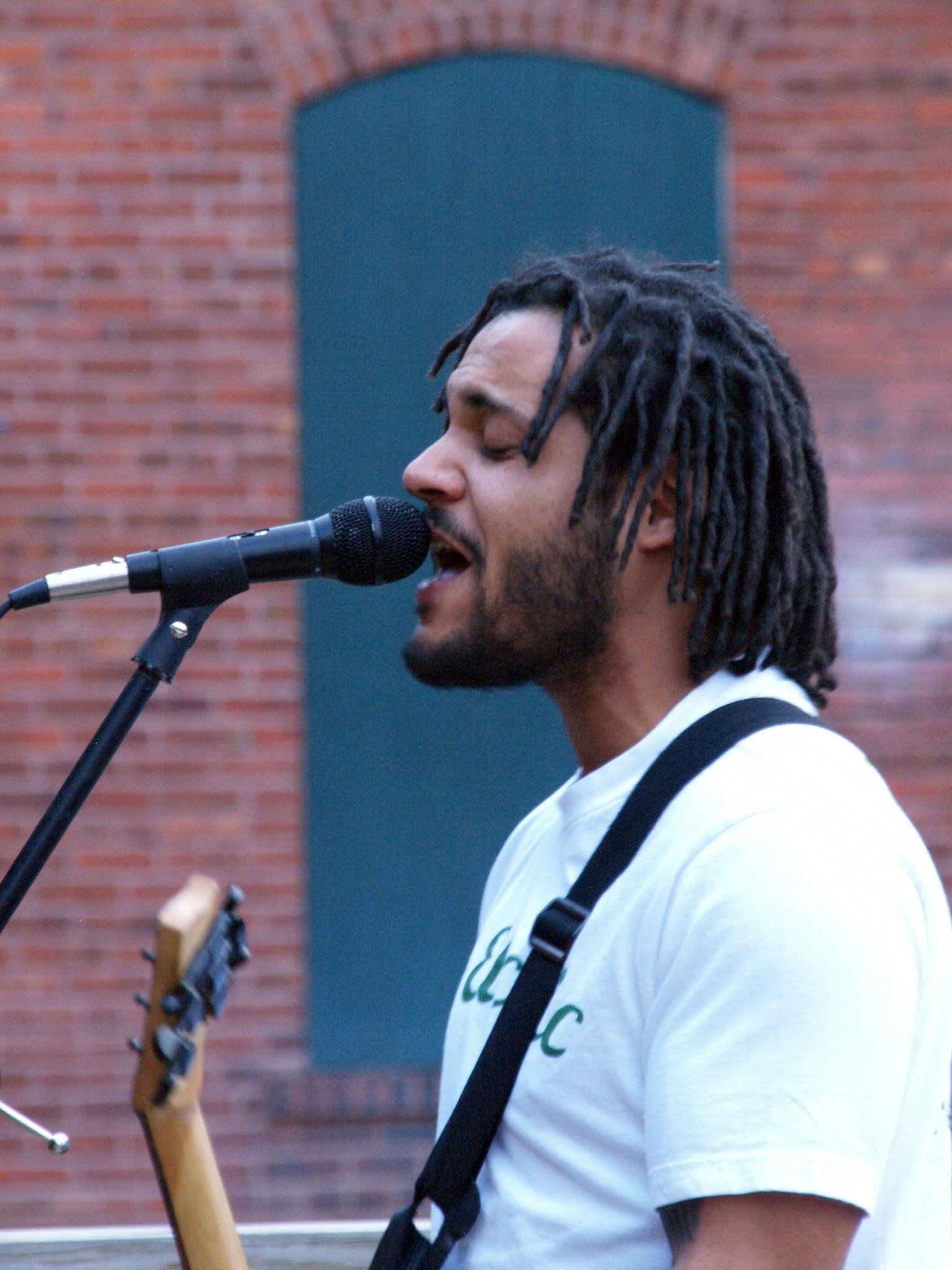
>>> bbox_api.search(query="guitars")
[124,868,252,1269]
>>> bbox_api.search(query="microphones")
[10,496,432,610]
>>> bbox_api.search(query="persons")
[370,244,952,1269]
